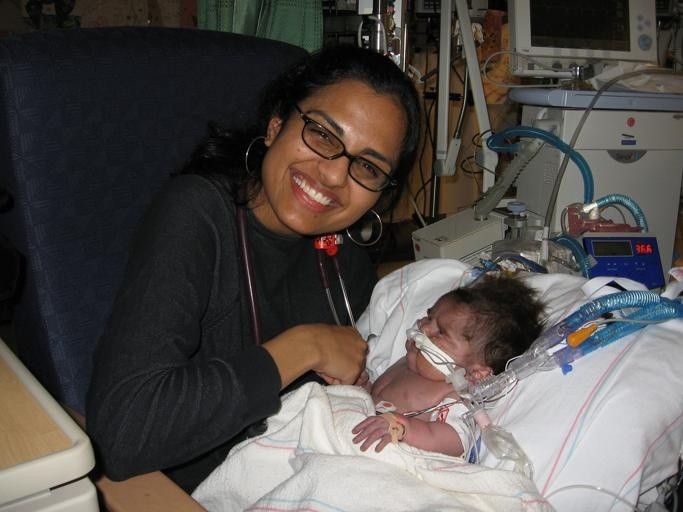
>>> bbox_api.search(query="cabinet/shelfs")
[513,106,682,295]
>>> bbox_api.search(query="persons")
[314,273,553,459]
[84,36,422,494]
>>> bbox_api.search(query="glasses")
[293,101,394,195]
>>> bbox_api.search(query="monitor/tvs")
[509,0,658,79]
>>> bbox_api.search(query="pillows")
[356,257,683,511]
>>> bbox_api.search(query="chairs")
[1,25,408,512]
